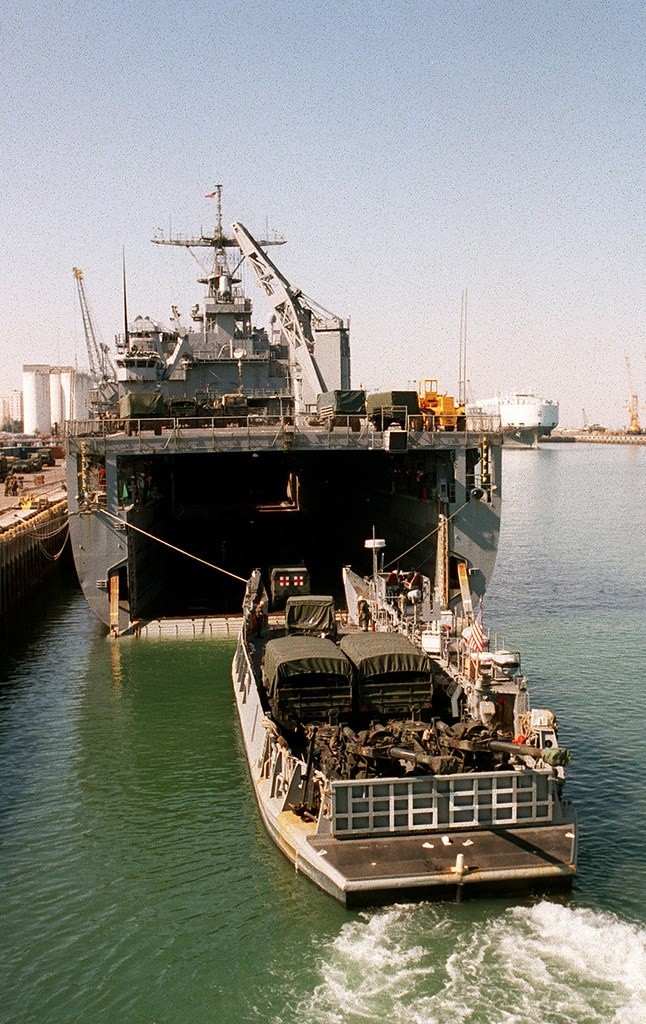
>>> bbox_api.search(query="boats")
[227,491,579,908]
[50,182,509,655]
[460,391,561,445]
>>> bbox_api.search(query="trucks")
[314,388,367,431]
[366,391,417,432]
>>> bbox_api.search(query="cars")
[0,447,54,484]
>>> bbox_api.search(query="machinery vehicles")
[409,378,469,433]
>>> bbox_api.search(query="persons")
[3,476,23,496]
[358,567,422,633]
